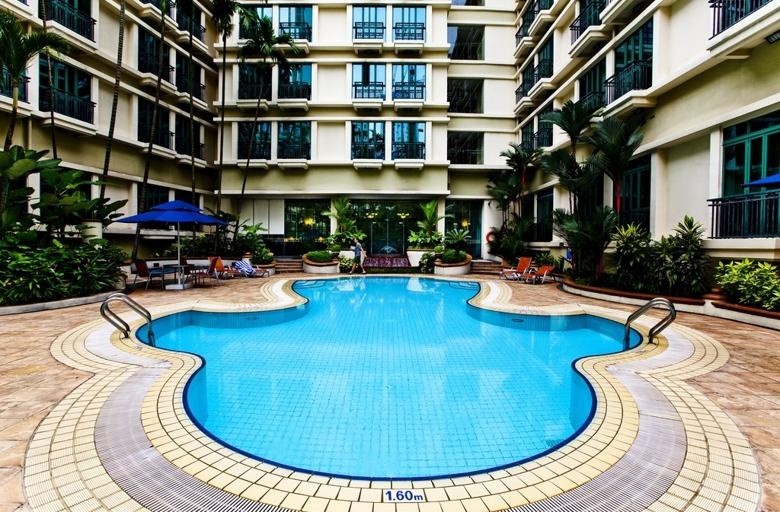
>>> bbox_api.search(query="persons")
[347,237,369,275]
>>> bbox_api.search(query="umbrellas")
[113,199,230,285]
[741,174,780,189]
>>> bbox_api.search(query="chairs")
[499,256,558,284]
[131,255,269,291]
